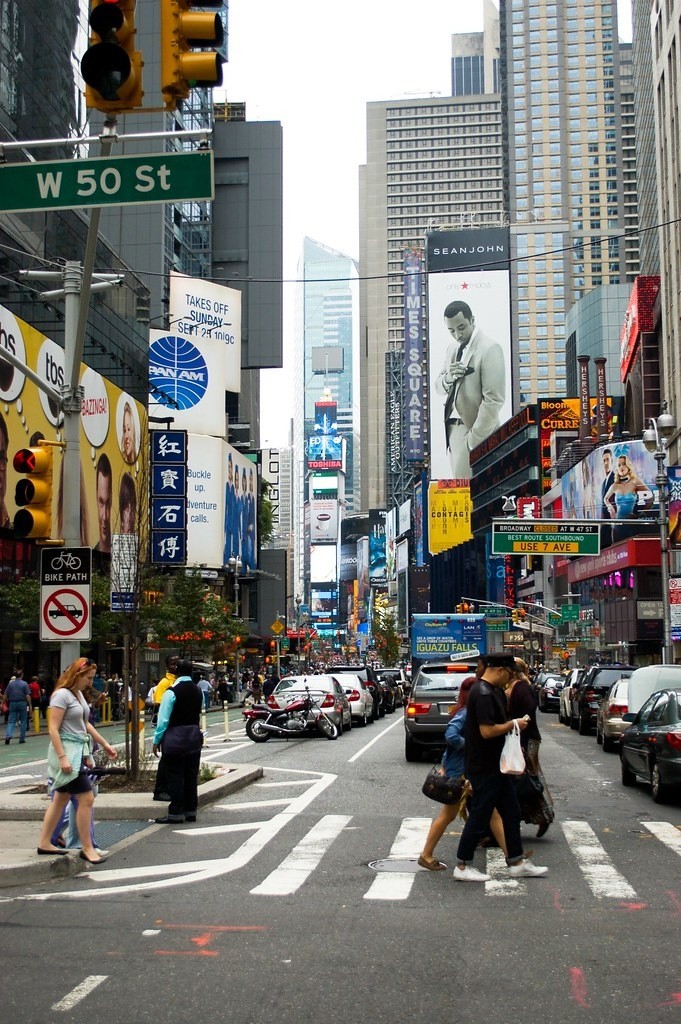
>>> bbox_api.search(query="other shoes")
[37,846,68,855]
[505,850,535,864]
[155,817,182,824]
[5,737,10,744]
[95,848,108,856]
[79,849,107,864]
[417,855,446,871]
[19,740,25,743]
[482,839,500,848]
[537,812,555,837]
[186,816,196,821]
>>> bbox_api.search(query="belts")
[447,418,464,424]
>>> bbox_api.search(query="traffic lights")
[80,0,140,108]
[161,0,228,102]
[519,609,526,623]
[13,442,61,542]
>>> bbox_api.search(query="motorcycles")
[242,677,339,743]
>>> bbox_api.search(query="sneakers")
[453,864,491,882]
[507,859,549,877]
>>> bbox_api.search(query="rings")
[453,375,458,380]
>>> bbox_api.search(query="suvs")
[325,665,386,719]
[573,661,639,734]
[403,659,480,761]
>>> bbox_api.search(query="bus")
[410,613,488,681]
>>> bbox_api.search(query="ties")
[444,343,466,421]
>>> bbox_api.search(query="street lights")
[641,399,677,664]
[296,593,303,675]
[227,555,242,703]
[456,604,462,613]
[511,609,518,623]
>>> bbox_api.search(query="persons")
[0,669,32,745]
[40,688,50,720]
[28,675,42,722]
[152,654,181,800]
[598,449,653,520]
[199,655,412,709]
[225,452,258,571]
[92,453,138,554]
[36,658,116,864]
[416,649,556,883]
[153,659,205,824]
[121,402,138,463]
[88,669,133,721]
[434,298,507,479]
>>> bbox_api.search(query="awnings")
[193,661,214,670]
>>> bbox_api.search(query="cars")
[595,677,631,753]
[530,668,584,725]
[267,674,375,736]
[373,664,412,715]
[617,687,681,803]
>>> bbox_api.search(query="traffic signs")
[637,601,665,619]
[561,604,579,622]
[273,635,284,639]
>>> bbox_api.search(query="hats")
[485,653,524,672]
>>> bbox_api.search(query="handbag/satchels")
[499,719,526,776]
[160,725,203,757]
[422,767,465,805]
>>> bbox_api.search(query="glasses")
[504,667,513,676]
